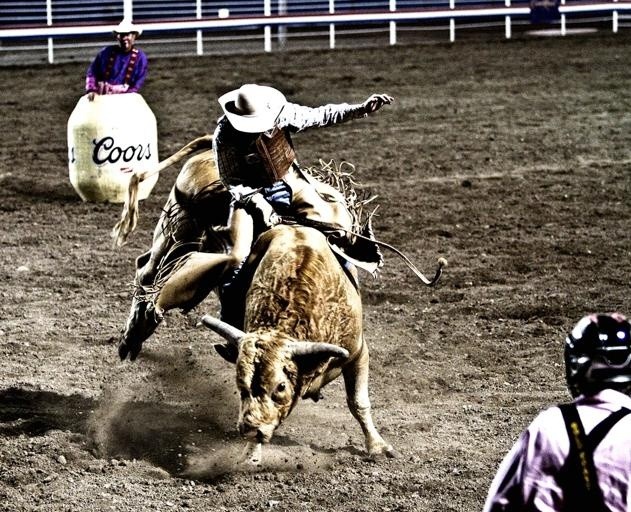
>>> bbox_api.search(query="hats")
[217,82,290,133]
[112,19,143,36]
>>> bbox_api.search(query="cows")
[112,135,399,463]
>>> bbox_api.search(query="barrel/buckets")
[67,91,161,204]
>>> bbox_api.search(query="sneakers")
[214,344,238,363]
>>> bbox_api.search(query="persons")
[146,82,395,316]
[85,21,148,101]
[483,312,631,512]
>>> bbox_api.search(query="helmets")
[565,312,631,398]
